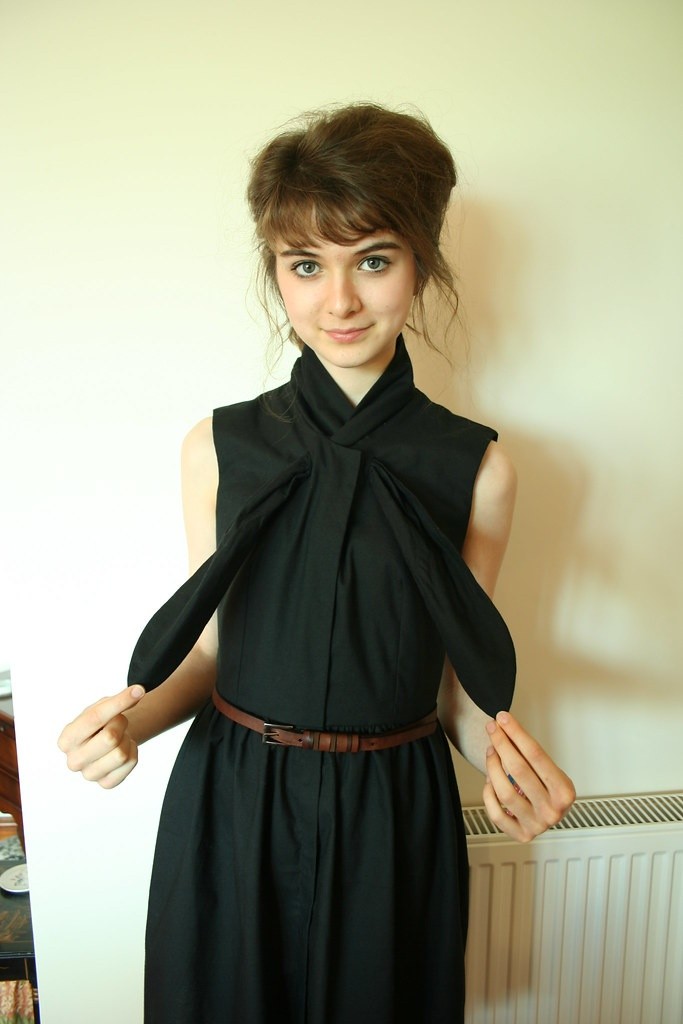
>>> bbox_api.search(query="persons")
[58,101,576,1024]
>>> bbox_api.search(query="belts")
[212,686,438,753]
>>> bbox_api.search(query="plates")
[0,864,29,893]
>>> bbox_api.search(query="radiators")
[462,793,683,1024]
